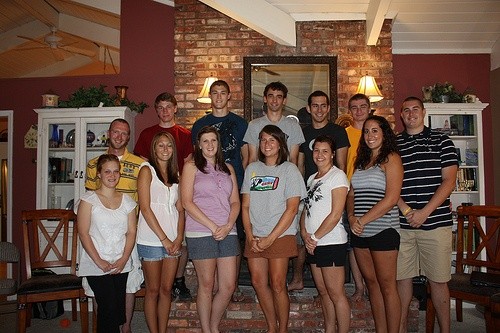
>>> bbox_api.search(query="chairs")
[0,208,88,333]
[426,205,500,333]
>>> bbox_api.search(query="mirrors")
[243,56,338,124]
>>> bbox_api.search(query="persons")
[240,125,309,333]
[191,80,248,303]
[135,132,185,333]
[346,93,372,303]
[82,118,147,333]
[299,135,351,333]
[388,97,458,333]
[347,115,404,333]
[288,90,351,309]
[133,92,194,300]
[73,154,137,333]
[181,126,241,333]
[243,81,305,304]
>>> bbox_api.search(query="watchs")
[311,235,318,241]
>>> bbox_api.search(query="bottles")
[444,119,449,128]
[51,165,58,183]
[87,129,95,146]
[51,125,64,146]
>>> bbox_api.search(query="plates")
[97,130,110,147]
[67,129,75,147]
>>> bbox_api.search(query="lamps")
[356,75,384,102]
[197,76,218,115]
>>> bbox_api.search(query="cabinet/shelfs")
[422,103,489,309]
[33,107,138,311]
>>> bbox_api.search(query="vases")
[41,94,60,108]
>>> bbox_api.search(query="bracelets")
[347,215,353,221]
[160,236,167,241]
[403,207,412,216]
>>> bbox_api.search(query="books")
[451,226,480,252]
[456,168,479,191]
[48,157,73,183]
[450,115,475,136]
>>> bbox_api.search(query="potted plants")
[431,81,454,103]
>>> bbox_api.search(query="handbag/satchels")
[30,268,65,320]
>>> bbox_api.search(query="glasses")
[350,104,368,111]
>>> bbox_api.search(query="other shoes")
[171,276,192,302]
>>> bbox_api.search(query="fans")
[10,26,96,62]
